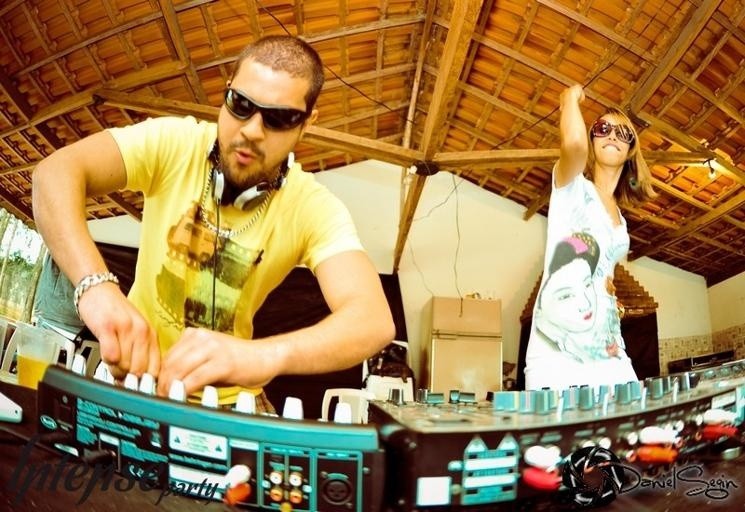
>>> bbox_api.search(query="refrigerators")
[421,296,505,401]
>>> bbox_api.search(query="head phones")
[206,135,294,212]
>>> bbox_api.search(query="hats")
[538,232,600,310]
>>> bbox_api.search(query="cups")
[16,321,58,391]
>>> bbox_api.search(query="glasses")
[591,119,634,144]
[224,88,308,131]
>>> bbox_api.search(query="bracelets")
[73,273,120,321]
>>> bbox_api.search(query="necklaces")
[200,165,281,236]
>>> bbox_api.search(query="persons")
[536,233,599,363]
[31,34,397,413]
[524,83,658,390]
